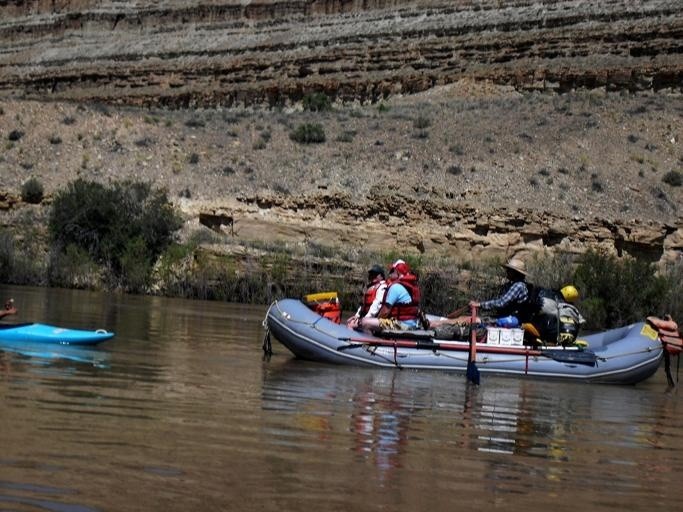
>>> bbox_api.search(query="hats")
[386,259,408,274]
[499,259,531,277]
[368,264,383,273]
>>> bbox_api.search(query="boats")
[2,343,113,372]
[265,290,683,390]
[0,321,113,345]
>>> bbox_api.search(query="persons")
[0,298,17,318]
[348,259,422,337]
[428,259,586,347]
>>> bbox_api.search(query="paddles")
[336,337,596,365]
[467,307,479,385]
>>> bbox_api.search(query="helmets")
[560,285,579,303]
